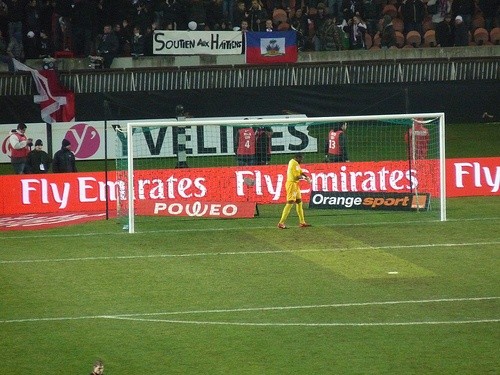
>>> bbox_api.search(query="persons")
[8,122,32,176]
[232,118,255,168]
[0,0,500,62]
[325,122,351,164]
[52,138,78,173]
[253,118,273,165]
[405,118,428,161]
[277,153,312,229]
[24,138,50,174]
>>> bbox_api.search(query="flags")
[244,31,298,65]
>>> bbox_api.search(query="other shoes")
[299,223,311,227]
[277,223,286,228]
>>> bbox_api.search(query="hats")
[35,139,43,146]
[455,15,462,21]
[62,139,70,146]
